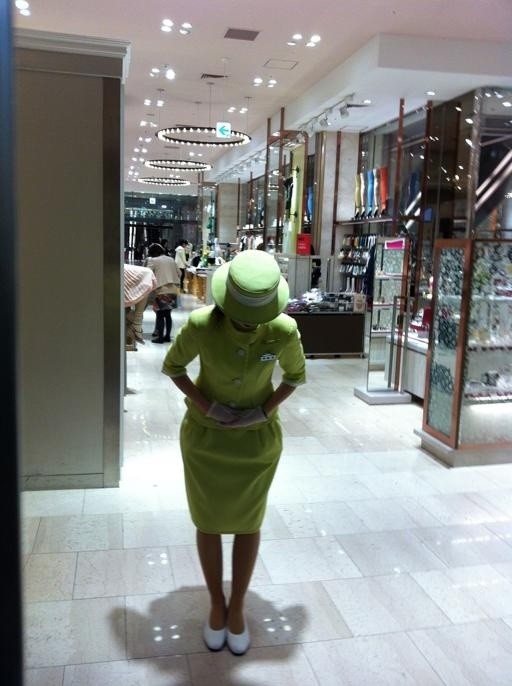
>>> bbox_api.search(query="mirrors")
[365,234,411,394]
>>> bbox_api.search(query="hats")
[211,250,290,324]
[409,306,431,331]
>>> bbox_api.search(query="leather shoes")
[151,330,171,343]
[203,610,250,656]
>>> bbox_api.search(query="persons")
[124,238,193,351]
[161,249,306,655]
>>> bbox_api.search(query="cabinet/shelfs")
[421,237,512,451]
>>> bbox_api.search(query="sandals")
[125,310,145,345]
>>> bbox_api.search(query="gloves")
[205,399,268,428]
